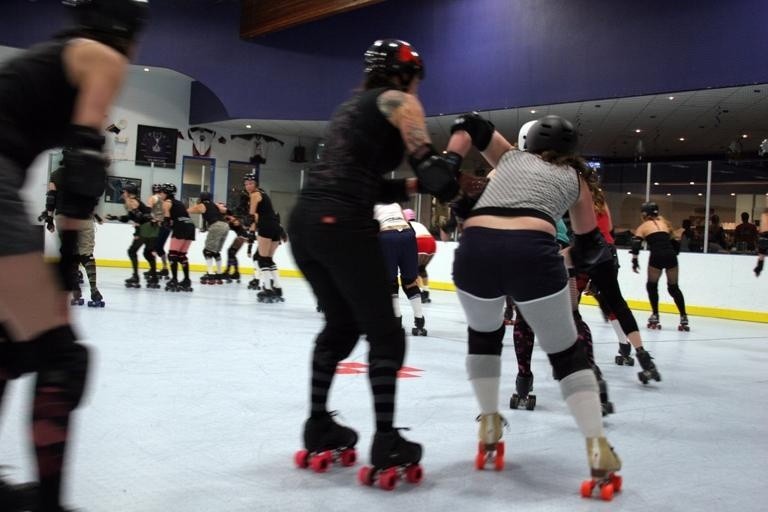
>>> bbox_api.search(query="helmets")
[121,182,177,196]
[517,115,578,155]
[62,1,149,41]
[239,173,258,201]
[199,191,212,201]
[402,208,415,220]
[363,37,425,79]
[640,201,659,216]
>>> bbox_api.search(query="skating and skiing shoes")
[200,272,240,284]
[677,314,690,331]
[296,410,358,473]
[69,271,104,307]
[504,308,516,325]
[474,413,508,470]
[598,378,614,414]
[248,279,284,303]
[511,372,536,409]
[617,342,659,384]
[581,437,622,501]
[411,289,431,336]
[359,428,423,491]
[124,268,193,292]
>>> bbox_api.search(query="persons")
[436,111,623,500]
[0,0,152,512]
[630,202,690,331]
[286,38,459,491]
[370,201,436,336]
[43,144,284,308]
[680,212,768,277]
[499,154,661,416]
